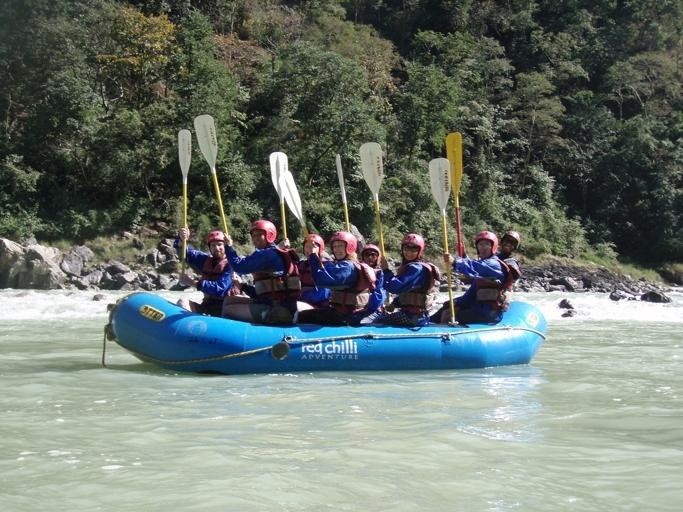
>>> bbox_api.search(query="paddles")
[193,115,233,250]
[359,143,385,257]
[429,158,455,323]
[268,151,289,242]
[447,133,463,259]
[279,172,308,239]
[337,155,350,233]
[177,129,191,286]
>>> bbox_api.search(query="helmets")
[329,231,357,255]
[360,243,382,266]
[207,230,224,244]
[400,233,425,260]
[302,234,324,257]
[501,230,520,249]
[249,220,277,243]
[475,231,499,255]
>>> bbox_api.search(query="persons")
[295,230,375,326]
[371,233,441,326]
[351,242,385,325]
[456,229,522,313]
[276,233,333,308]
[173,225,243,318]
[426,230,513,325]
[219,219,299,326]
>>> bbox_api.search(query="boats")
[108,290,550,371]
